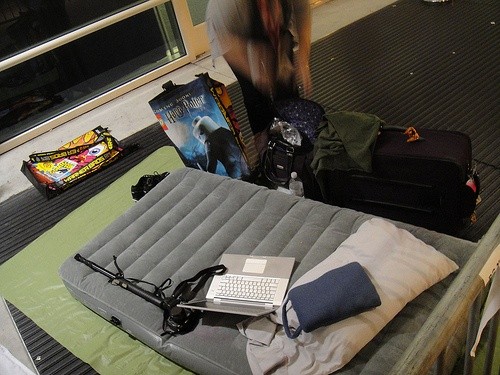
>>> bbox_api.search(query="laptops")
[176,252,295,318]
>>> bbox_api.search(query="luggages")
[257,125,480,233]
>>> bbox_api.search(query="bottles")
[289,172,304,198]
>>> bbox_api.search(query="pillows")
[237,218,459,375]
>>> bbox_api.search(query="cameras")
[166,302,202,332]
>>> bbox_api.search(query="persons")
[205,0,315,164]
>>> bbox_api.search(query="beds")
[61,167,477,375]
